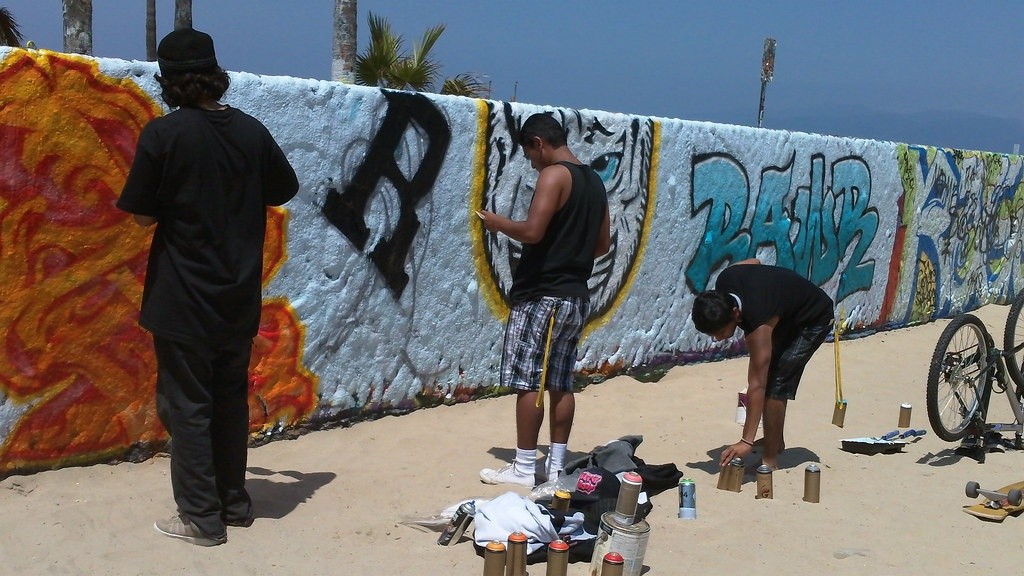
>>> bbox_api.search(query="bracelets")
[740,436,754,446]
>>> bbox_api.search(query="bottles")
[832,398,847,428]
[483,539,506,576]
[601,551,624,576]
[716,457,745,493]
[551,490,571,512]
[755,465,772,499]
[546,539,570,576]
[613,471,642,525]
[898,401,912,428]
[677,477,696,519]
[435,500,476,547]
[506,529,528,576]
[803,463,821,503]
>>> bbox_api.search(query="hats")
[157,27,217,80]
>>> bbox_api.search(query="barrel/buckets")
[735,392,763,428]
[588,511,651,576]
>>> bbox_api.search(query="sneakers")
[479,460,536,491]
[536,463,558,482]
[153,518,220,547]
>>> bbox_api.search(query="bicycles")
[926,286,1024,464]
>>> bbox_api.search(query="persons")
[117,27,299,548]
[477,112,612,489]
[690,259,835,470]
